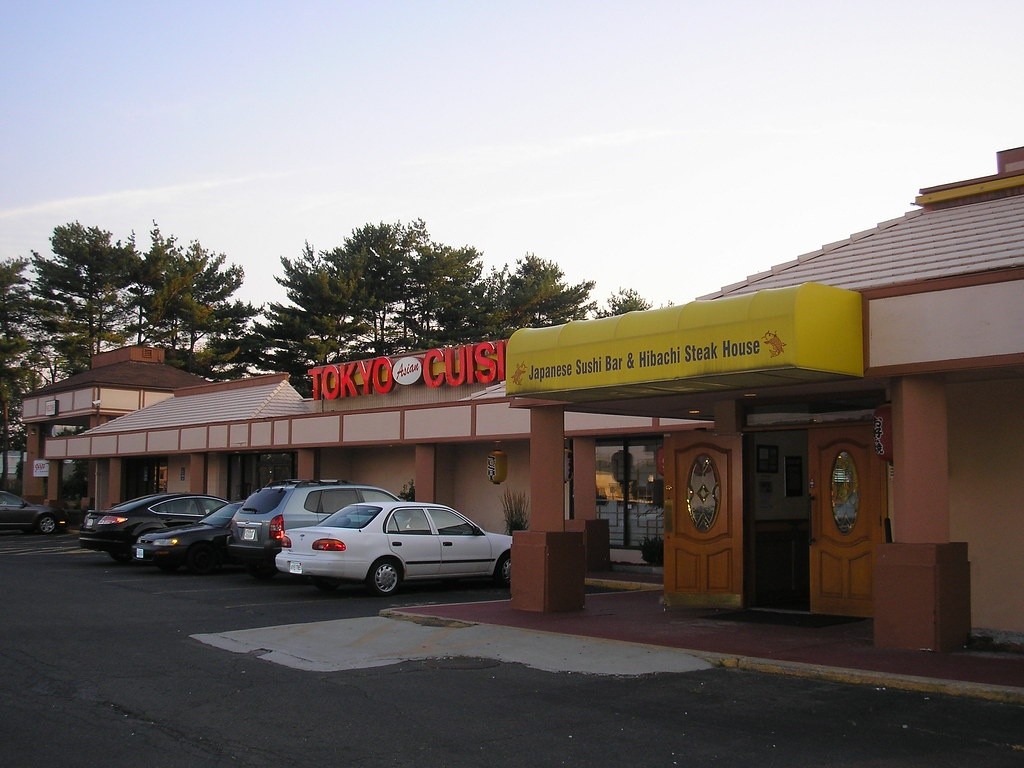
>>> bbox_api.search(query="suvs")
[226,483,420,569]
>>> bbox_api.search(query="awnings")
[505,282,864,401]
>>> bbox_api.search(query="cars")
[276,500,514,593]
[0,489,68,535]
[132,500,246,573]
[77,491,233,563]
[597,500,665,547]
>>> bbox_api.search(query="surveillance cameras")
[91,400,101,406]
